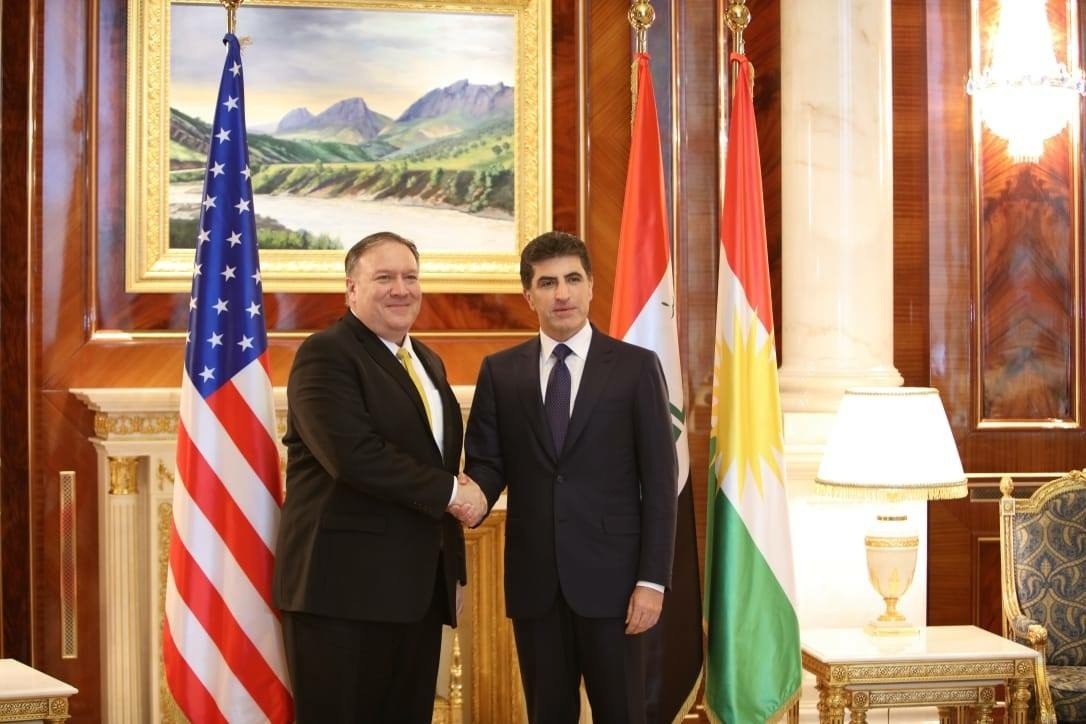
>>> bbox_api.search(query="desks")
[788,624,1039,724]
[0,656,77,724]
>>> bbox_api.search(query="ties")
[544,344,573,464]
[397,349,432,429]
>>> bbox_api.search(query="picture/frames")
[126,0,553,294]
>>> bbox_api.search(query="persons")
[270,232,482,724]
[450,231,679,724]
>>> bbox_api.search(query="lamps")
[813,385,967,637]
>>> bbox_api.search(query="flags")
[702,61,801,724]
[604,55,704,724]
[164,33,294,724]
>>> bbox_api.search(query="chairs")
[998,468,1086,724]
